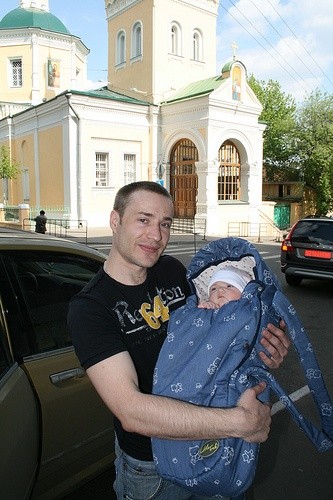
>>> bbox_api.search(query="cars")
[0,227,118,499]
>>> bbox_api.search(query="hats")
[207,266,252,297]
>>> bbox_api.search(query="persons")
[152,238,278,496]
[67,181,291,500]
[33,209,48,234]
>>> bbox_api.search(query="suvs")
[280,214,333,288]
